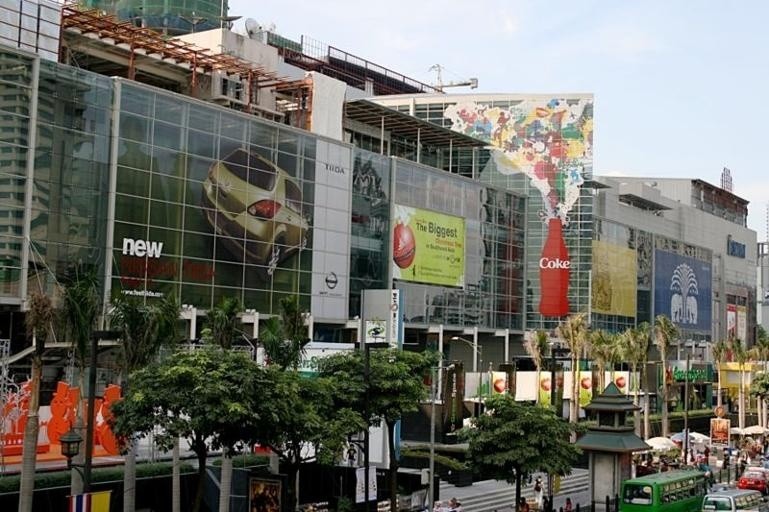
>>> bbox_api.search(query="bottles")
[539,217,570,318]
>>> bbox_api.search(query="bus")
[618,470,708,512]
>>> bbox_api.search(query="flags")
[491,370,631,411]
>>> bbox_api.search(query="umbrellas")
[671,425,769,442]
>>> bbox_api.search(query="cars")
[737,467,769,495]
[200,148,308,282]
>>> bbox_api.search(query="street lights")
[451,336,489,419]
[233,339,257,454]
[365,341,391,511]
[659,462,679,473]
[59,330,123,493]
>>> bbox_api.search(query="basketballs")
[393,225,415,268]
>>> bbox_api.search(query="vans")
[700,488,769,512]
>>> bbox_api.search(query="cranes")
[427,64,477,91]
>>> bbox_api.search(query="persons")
[532,474,545,507]
[344,444,357,467]
[433,501,444,512]
[520,496,529,512]
[641,435,769,487]
[447,498,463,512]
[564,497,573,511]
[249,480,279,511]
[542,495,549,511]
